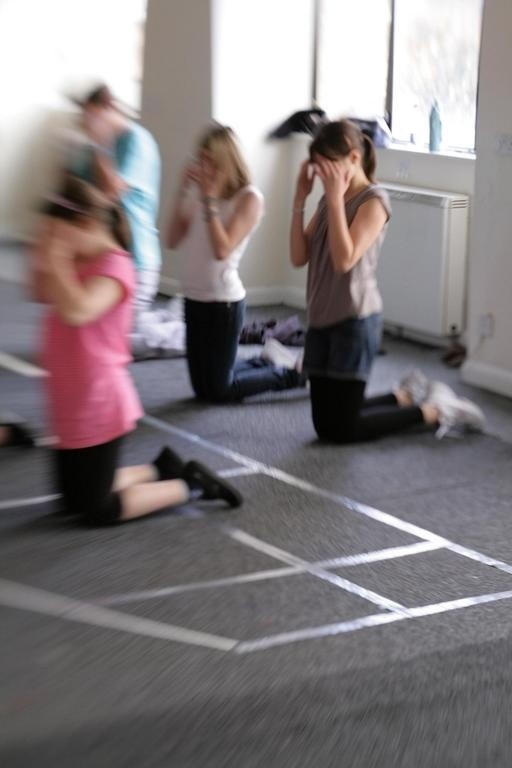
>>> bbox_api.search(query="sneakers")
[157,446,242,508]
[402,368,486,432]
[262,339,295,367]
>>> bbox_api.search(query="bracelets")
[199,195,218,206]
[291,206,305,216]
[201,208,220,222]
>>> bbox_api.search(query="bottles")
[429,104,444,152]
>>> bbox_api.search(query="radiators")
[376,181,470,339]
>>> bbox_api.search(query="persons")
[287,119,487,445]
[19,169,245,528]
[163,122,312,407]
[65,75,189,362]
